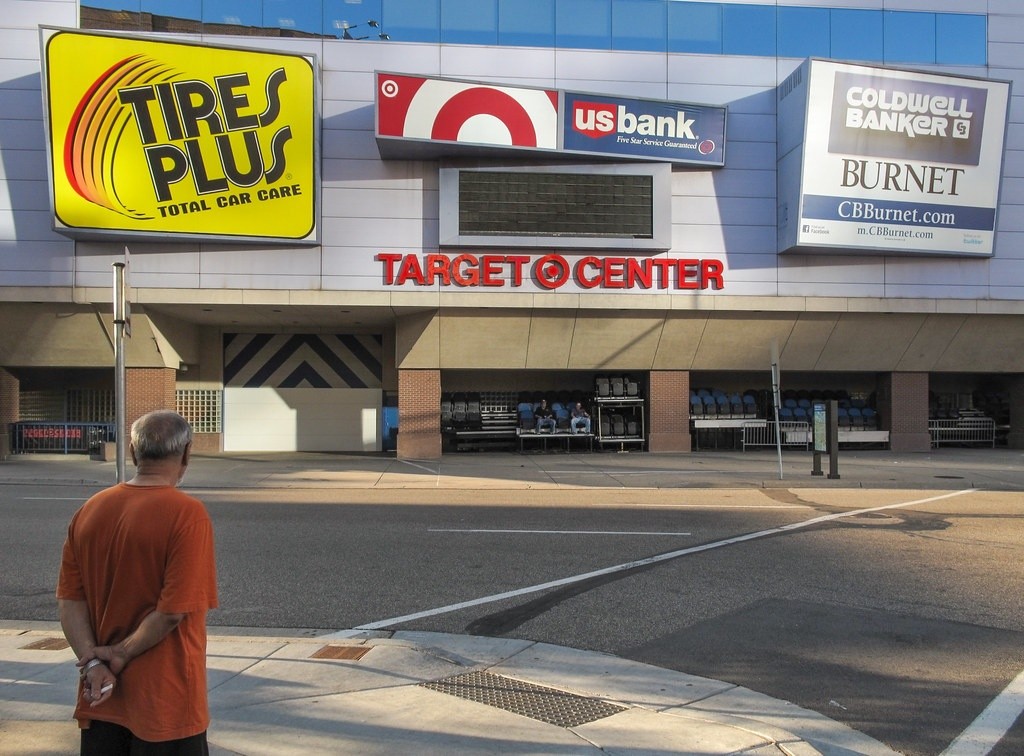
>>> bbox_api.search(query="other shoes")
[572,432,576,435]
[537,433,541,435]
[550,432,554,435]
[586,432,590,435]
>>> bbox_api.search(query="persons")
[56,411,218,756]
[535,400,556,435]
[571,402,591,435]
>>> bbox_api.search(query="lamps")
[343,21,379,39]
[360,34,390,40]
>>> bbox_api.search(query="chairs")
[688,387,878,431]
[442,392,485,431]
[520,375,640,438]
[930,392,962,422]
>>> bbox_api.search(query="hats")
[542,399,546,403]
[576,403,581,406]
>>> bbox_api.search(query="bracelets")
[83,658,103,674]
